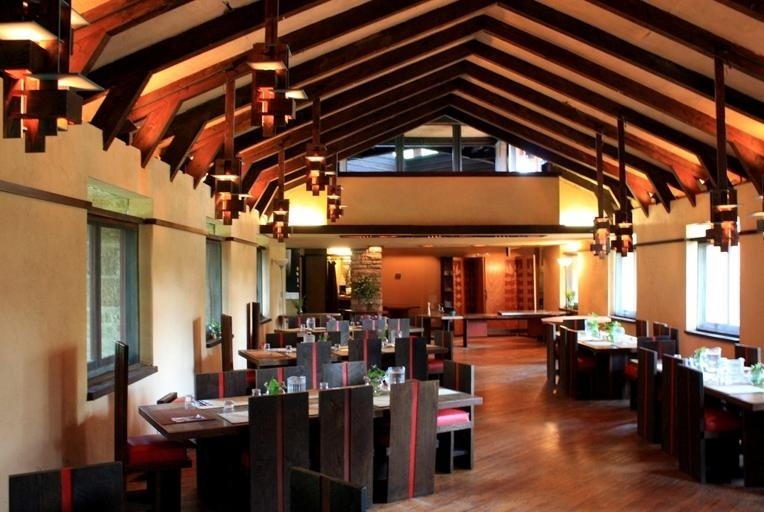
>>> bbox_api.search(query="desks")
[418,309,568,349]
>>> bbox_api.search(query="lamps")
[707,56,738,253]
[1,0,75,154]
[593,116,633,259]
[207,0,342,243]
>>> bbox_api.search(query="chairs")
[545,319,763,484]
[8,302,474,511]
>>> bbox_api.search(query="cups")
[184,317,405,416]
[583,316,743,386]
